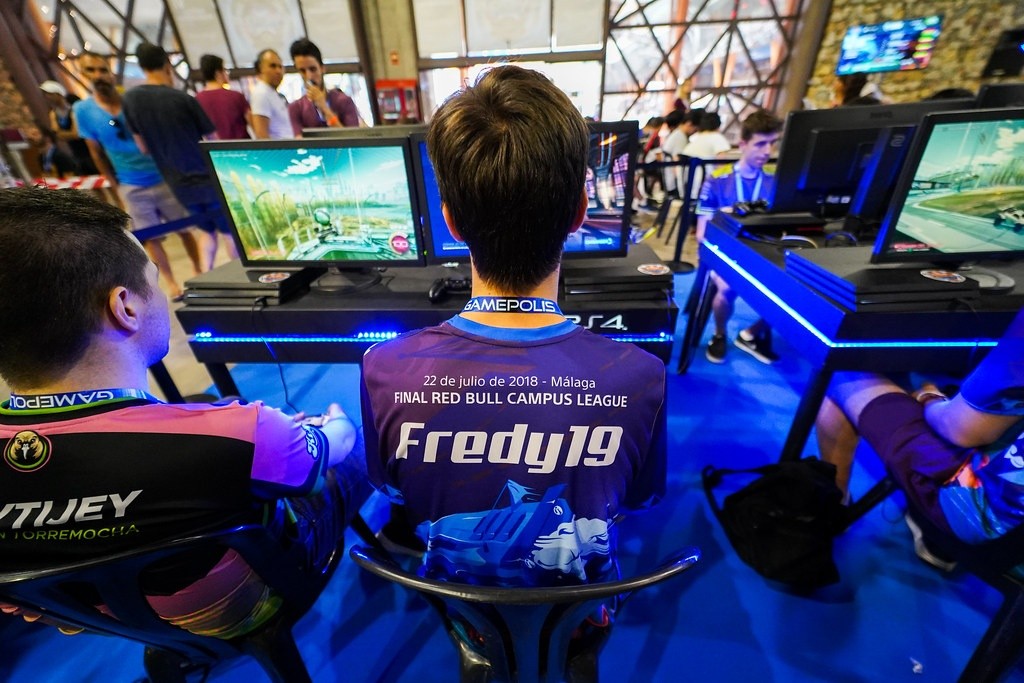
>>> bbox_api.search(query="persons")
[288,38,360,139]
[831,72,881,106]
[119,42,239,273]
[695,110,779,365]
[194,54,252,140]
[37,80,100,175]
[246,49,293,140]
[359,64,668,588]
[632,107,731,215]
[69,50,204,302]
[0,185,357,641]
[815,304,1024,572]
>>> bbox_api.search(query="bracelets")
[916,392,948,401]
[327,116,337,126]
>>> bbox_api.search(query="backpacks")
[702,455,843,597]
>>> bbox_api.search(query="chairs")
[0,521,391,683]
[653,150,706,244]
[850,475,1023,683]
[351,545,700,683]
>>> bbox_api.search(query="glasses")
[109,118,127,140]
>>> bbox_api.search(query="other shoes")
[628,226,656,244]
[904,507,960,572]
[705,331,728,365]
[733,331,782,365]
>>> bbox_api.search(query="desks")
[174,242,679,398]
[676,217,1024,460]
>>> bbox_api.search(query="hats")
[39,80,67,98]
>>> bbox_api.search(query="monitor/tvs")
[199,119,638,294]
[834,14,942,78]
[769,84,1024,293]
